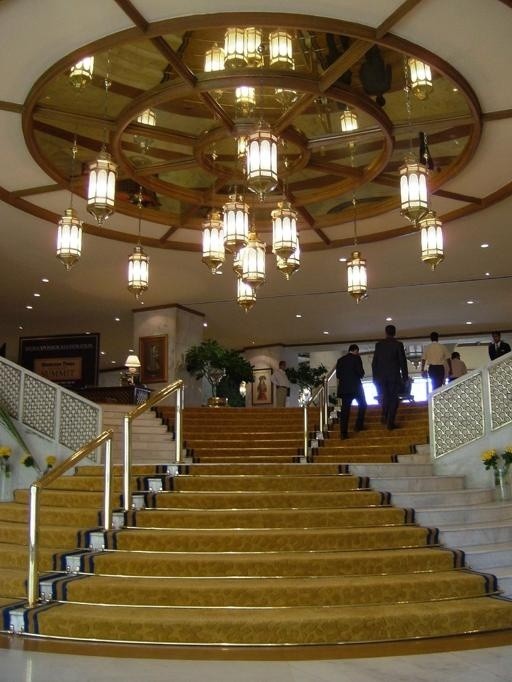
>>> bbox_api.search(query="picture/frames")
[138,332,169,384]
[251,367,275,405]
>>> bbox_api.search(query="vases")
[1,460,16,502]
[492,466,511,502]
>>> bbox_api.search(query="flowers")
[480,444,512,486]
[20,450,58,477]
[1,445,15,479]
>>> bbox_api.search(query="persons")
[488,331,511,360]
[421,332,452,391]
[372,326,408,429]
[270,361,290,408]
[336,345,367,439]
[448,352,467,382]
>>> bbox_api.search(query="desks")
[72,386,158,406]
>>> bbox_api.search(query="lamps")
[68,55,94,92]
[408,57,433,101]
[86,72,118,225]
[203,125,300,312]
[55,21,446,316]
[134,108,157,154]
[127,186,150,301]
[56,133,84,274]
[419,131,445,272]
[398,94,429,228]
[204,26,295,73]
[236,86,257,117]
[340,106,359,148]
[346,193,367,304]
[124,355,142,386]
[275,88,296,117]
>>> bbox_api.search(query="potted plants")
[183,333,233,407]
